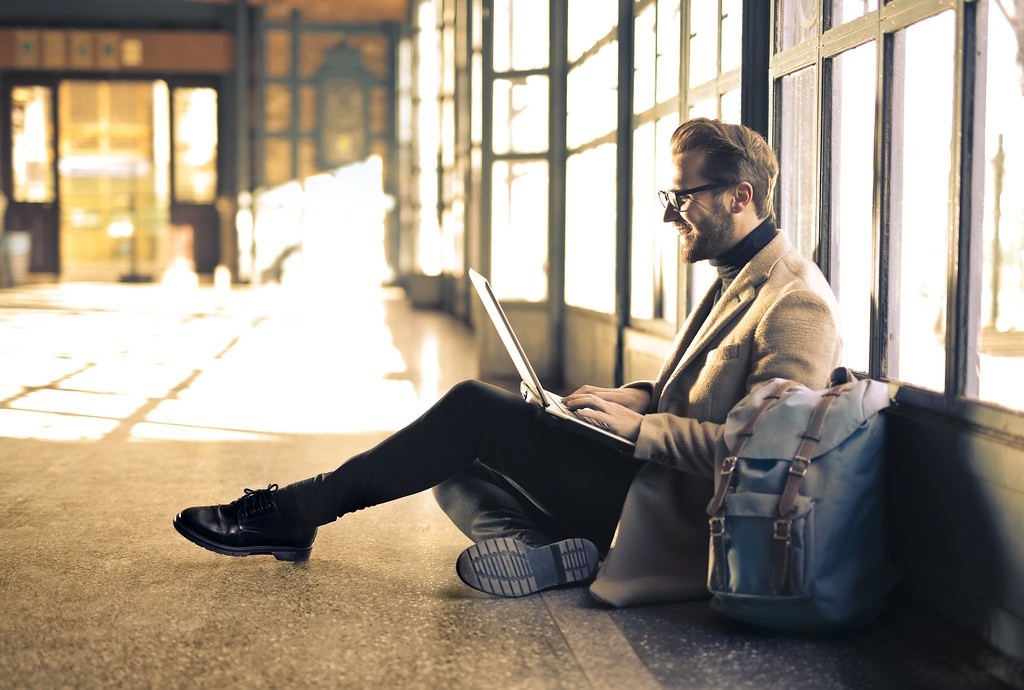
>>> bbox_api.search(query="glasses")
[658,183,728,212]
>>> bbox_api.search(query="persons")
[172,118,844,599]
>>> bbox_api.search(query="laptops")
[466,268,639,452]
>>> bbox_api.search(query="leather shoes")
[172,482,314,561]
[454,536,600,599]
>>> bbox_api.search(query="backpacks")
[705,377,895,636]
